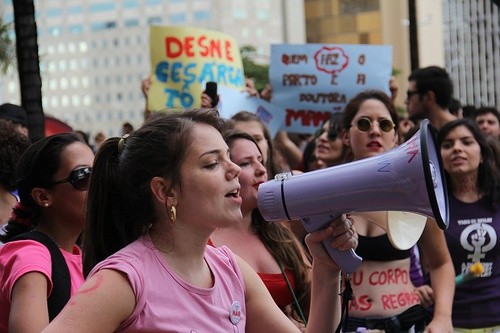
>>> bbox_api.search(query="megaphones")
[258,122,449,274]
[353,210,427,250]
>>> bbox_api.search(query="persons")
[274,128,305,172]
[405,66,462,143]
[397,118,416,146]
[315,114,352,173]
[142,77,212,112]
[0,131,96,333]
[409,119,500,333]
[207,131,313,333]
[0,121,30,226]
[43,110,359,333]
[225,111,275,184]
[472,108,500,139]
[0,104,29,139]
[443,100,464,119]
[334,90,455,333]
[301,138,327,172]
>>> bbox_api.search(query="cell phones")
[206,82,217,107]
